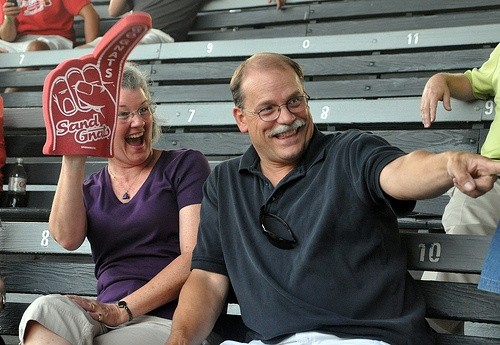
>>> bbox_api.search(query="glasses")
[259,198,298,250]
[243,91,310,122]
[117,106,157,121]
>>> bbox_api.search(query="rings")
[98,314,101,320]
[92,303,94,310]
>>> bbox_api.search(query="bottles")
[6,157,27,208]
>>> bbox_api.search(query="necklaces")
[108,166,144,199]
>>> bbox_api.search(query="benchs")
[0,0,500,345]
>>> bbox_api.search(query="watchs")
[118,301,133,321]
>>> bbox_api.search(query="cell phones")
[7,0,18,6]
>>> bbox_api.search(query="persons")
[420,42,500,333]
[166,52,500,345]
[76,0,209,65]
[0,0,100,93]
[19,61,212,345]
